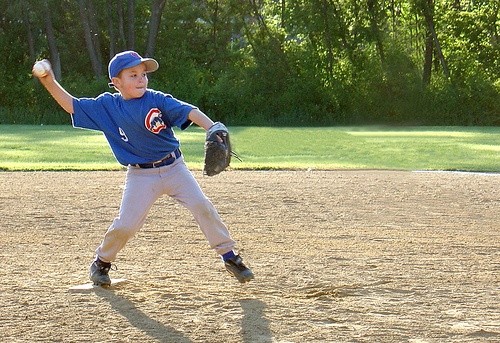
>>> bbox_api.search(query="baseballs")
[33,61,50,77]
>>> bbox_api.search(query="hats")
[108,50,159,79]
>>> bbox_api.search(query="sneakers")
[224,254,254,280]
[90,257,111,285]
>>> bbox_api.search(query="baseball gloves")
[203,121,242,176]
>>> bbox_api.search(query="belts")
[131,148,182,168]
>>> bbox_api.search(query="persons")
[35,50,253,287]
